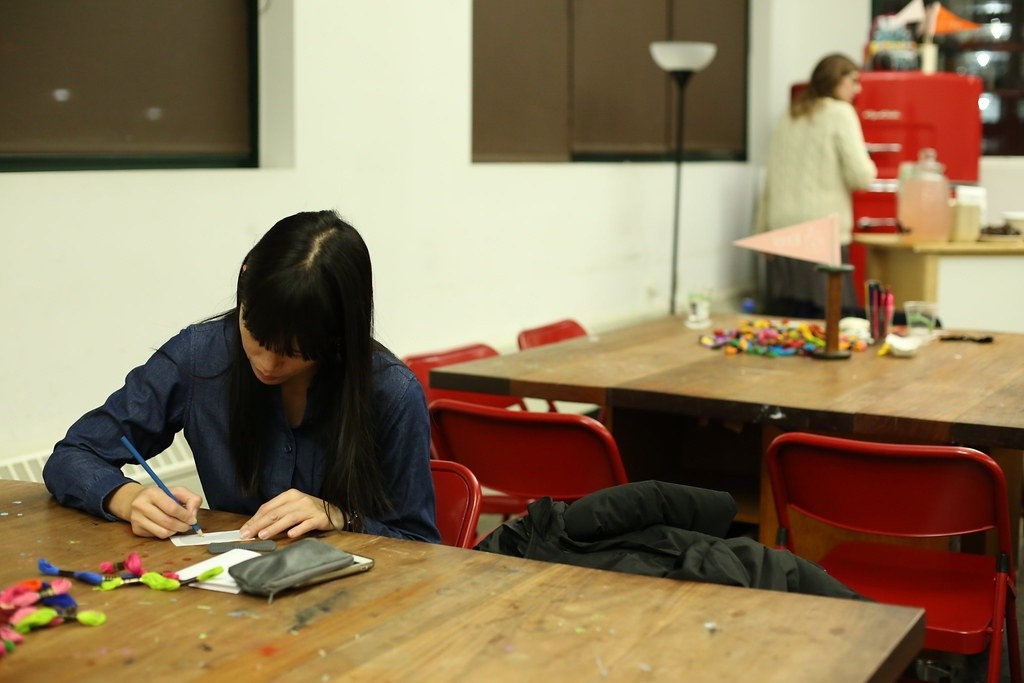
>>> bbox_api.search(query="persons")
[758,53,879,320]
[41,209,443,545]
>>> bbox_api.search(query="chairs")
[517,321,615,441]
[403,344,631,552]
[764,429,1024,682]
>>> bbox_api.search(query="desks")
[0,476,928,683]
[845,233,1023,332]
[427,308,1023,562]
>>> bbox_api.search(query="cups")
[955,204,980,244]
[865,304,896,342]
[903,299,936,334]
[684,290,711,323]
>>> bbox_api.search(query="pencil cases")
[227,536,353,598]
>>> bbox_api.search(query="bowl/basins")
[1003,212,1024,236]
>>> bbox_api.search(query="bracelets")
[337,505,349,530]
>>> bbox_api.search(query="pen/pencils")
[120,434,204,539]
[863,279,894,343]
[942,334,995,345]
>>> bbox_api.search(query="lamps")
[650,41,717,316]
